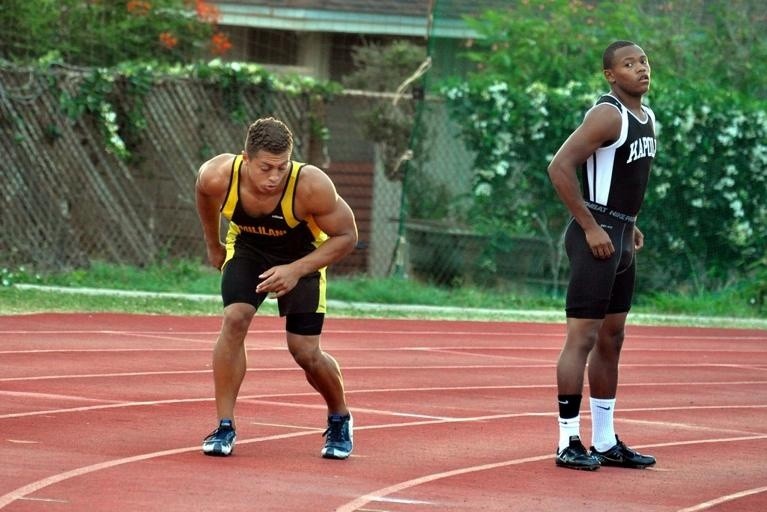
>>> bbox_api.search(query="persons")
[194,118,358,459]
[545,40,658,470]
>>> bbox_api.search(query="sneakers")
[588,434,657,469]
[320,411,354,459]
[201,419,238,455]
[555,435,602,472]
